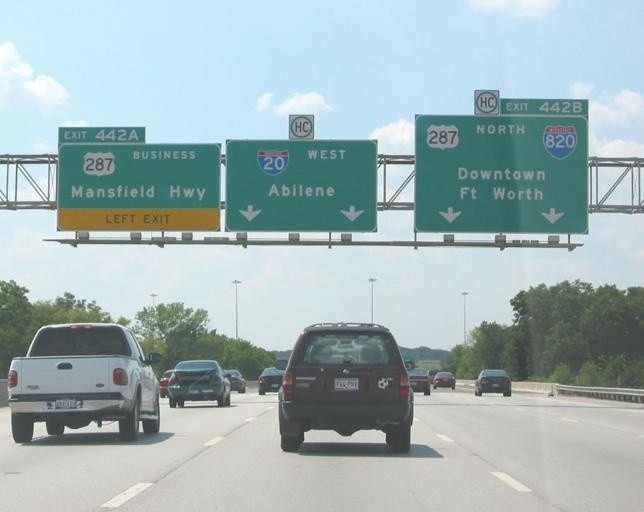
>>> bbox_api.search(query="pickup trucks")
[6,322,160,443]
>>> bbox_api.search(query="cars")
[407,369,513,397]
[160,359,286,407]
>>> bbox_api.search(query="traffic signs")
[224,139,378,232]
[57,145,220,232]
[414,114,588,234]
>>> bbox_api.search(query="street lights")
[367,278,379,326]
[231,279,242,341]
[150,293,158,305]
[460,290,469,346]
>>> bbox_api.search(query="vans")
[277,321,414,452]
